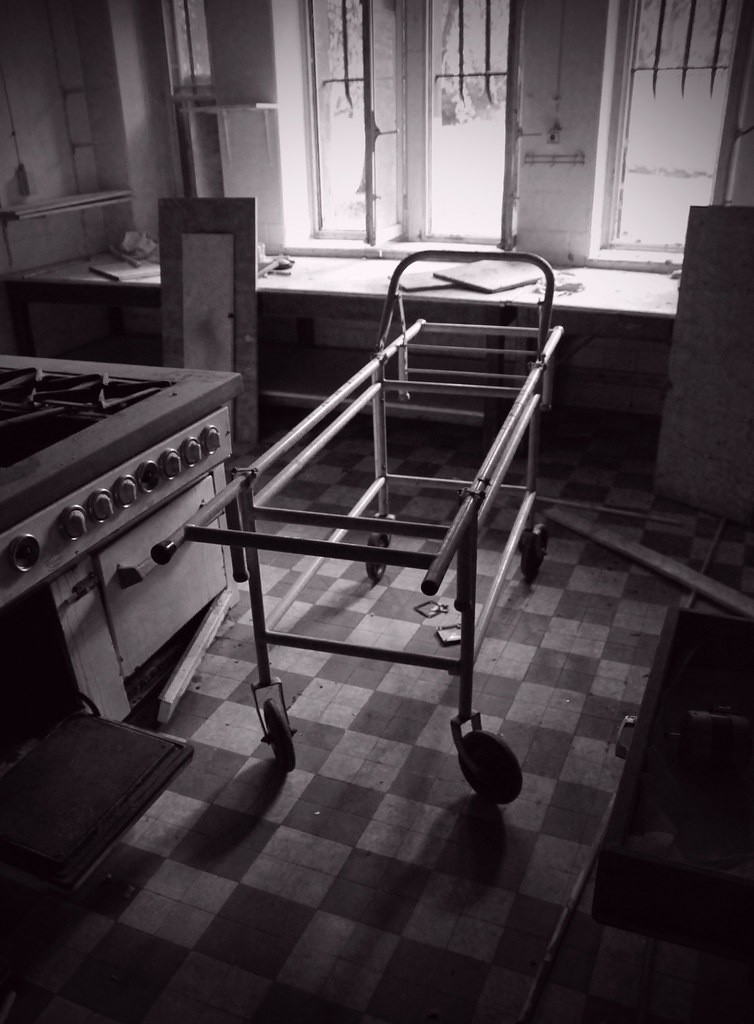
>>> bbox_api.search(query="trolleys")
[150,252,562,808]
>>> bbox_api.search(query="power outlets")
[546,132,561,145]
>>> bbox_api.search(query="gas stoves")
[0,351,245,611]
